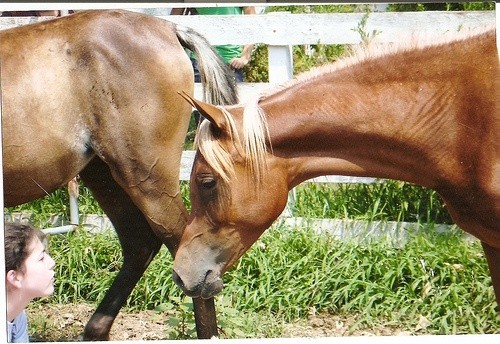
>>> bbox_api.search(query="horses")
[170,22,499,310]
[0,10,239,340]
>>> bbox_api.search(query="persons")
[4,221,56,342]
[170,7,258,128]
